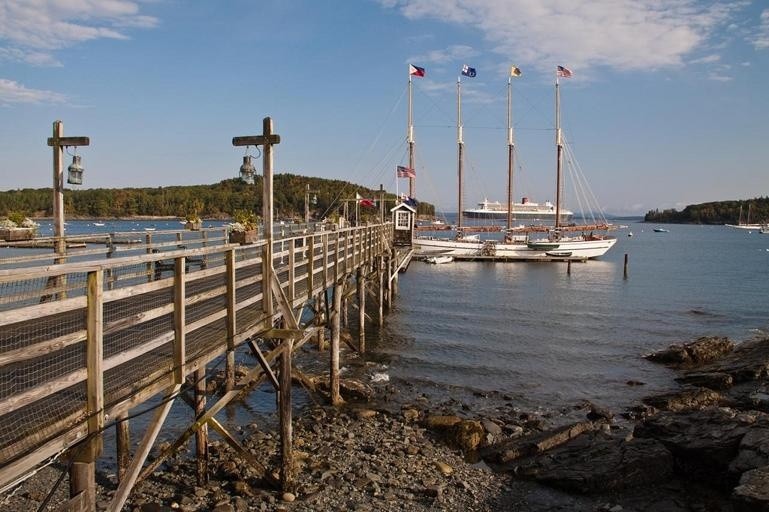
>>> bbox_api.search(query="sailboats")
[393,64,619,261]
[726,204,769,234]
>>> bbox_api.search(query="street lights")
[303,183,320,229]
[45,119,92,302]
[233,115,281,316]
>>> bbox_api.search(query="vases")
[228,228,258,244]
[185,222,201,230]
[3,228,36,241]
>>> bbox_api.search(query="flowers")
[0,211,40,228]
[186,214,203,223]
[224,209,257,234]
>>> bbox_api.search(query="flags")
[459,64,477,77]
[555,64,573,80]
[355,192,376,209]
[395,163,418,181]
[509,63,523,78]
[409,63,425,78]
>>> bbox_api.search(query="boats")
[62,213,294,233]
[461,198,576,223]
